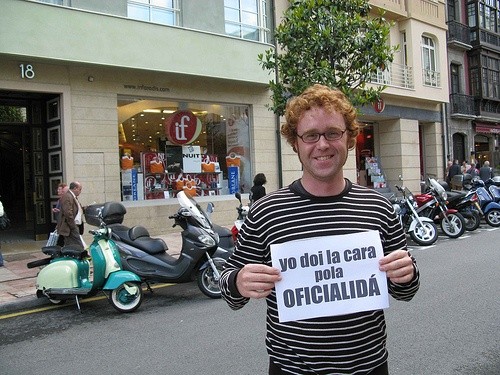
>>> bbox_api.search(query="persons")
[247,173,267,206]
[218,84,420,375]
[51,183,69,229]
[57,181,84,249]
[444,158,495,186]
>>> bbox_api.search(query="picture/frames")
[50,201,57,223]
[48,125,61,149]
[46,96,61,123]
[48,151,62,173]
[50,176,62,199]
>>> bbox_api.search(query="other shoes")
[45,229,59,247]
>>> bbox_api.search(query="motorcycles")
[412,177,466,239]
[382,174,438,247]
[437,175,500,231]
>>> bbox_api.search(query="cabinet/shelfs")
[147,172,221,198]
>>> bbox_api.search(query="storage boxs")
[85,201,126,226]
[450,173,471,188]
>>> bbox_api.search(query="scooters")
[84,189,233,298]
[34,205,143,314]
[230,192,253,242]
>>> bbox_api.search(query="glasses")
[296,127,347,143]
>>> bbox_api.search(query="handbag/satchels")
[0,212,11,231]
